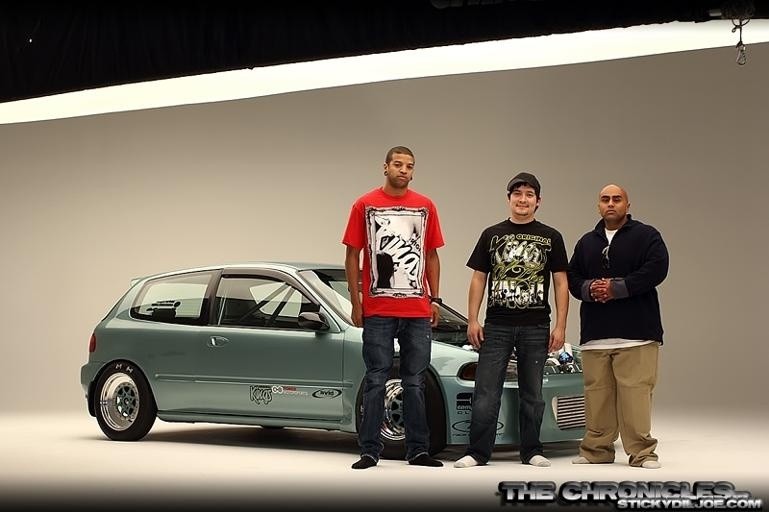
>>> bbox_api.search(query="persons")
[450,171,570,467]
[566,183,669,470]
[341,143,444,467]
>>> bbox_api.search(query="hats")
[507,171,541,198]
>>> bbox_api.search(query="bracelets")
[429,300,441,308]
[430,297,443,304]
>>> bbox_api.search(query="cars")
[81,261,617,448]
